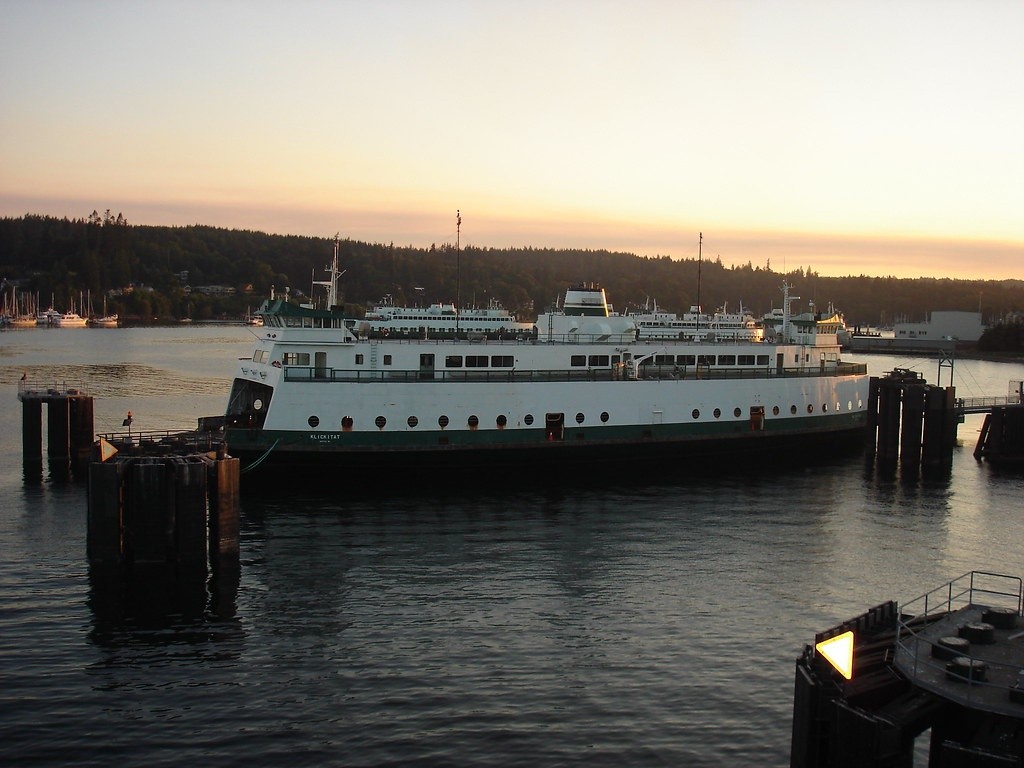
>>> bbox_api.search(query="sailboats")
[2,285,118,328]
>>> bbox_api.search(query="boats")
[163,209,886,471]
